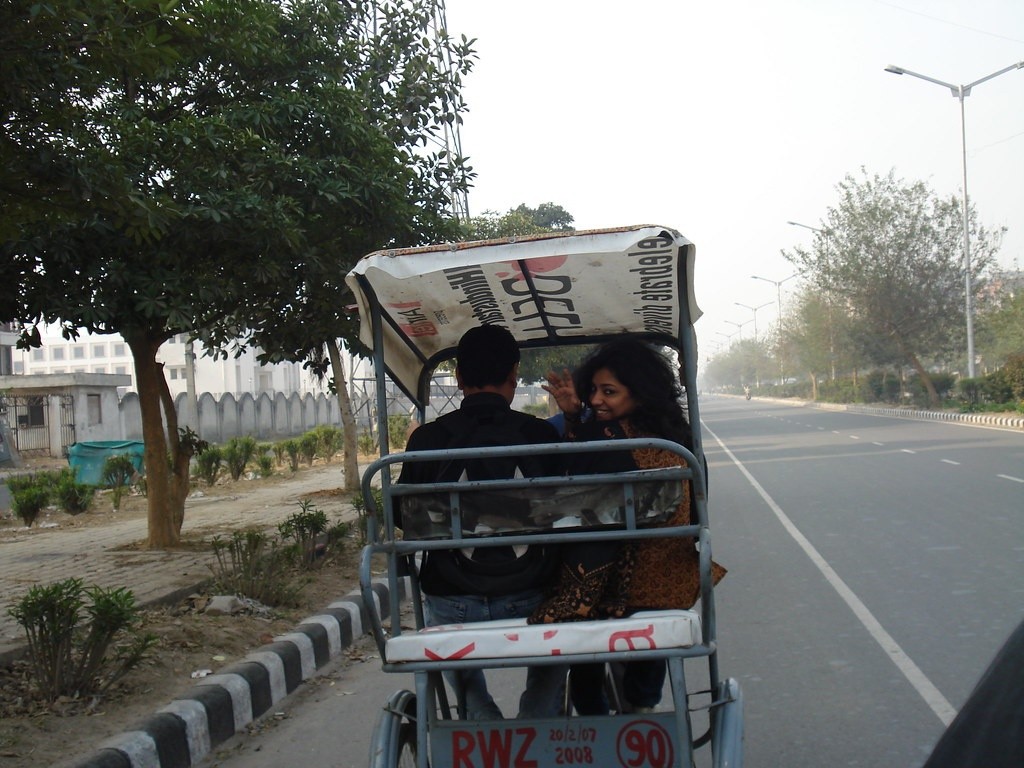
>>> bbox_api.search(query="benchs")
[382,602,705,665]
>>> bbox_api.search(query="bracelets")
[564,413,579,423]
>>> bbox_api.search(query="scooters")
[745,392,751,400]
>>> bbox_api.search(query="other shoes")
[607,673,656,714]
[470,706,504,720]
[518,709,554,719]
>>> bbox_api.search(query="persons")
[527,335,728,717]
[391,324,568,720]
[745,387,751,397]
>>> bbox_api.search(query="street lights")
[751,269,808,386]
[735,301,775,387]
[787,222,848,381]
[723,320,752,341]
[882,61,1024,379]
[716,332,740,351]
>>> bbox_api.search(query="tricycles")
[345,224,744,768]
